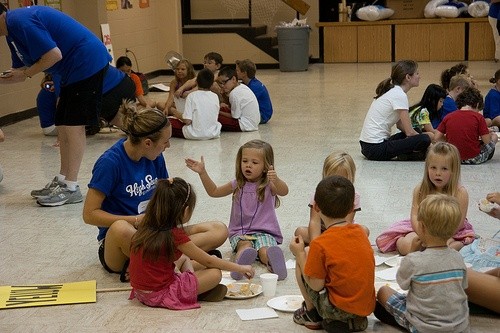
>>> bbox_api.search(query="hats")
[490,70,500,84]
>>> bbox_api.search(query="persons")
[128,177,255,310]
[290,176,375,331]
[488,0,500,60]
[375,142,476,255]
[0,1,112,206]
[396,65,500,164]
[295,151,370,244]
[464,191,500,316]
[184,139,288,280]
[375,194,471,333]
[83,99,228,274]
[37,52,273,139]
[359,60,431,162]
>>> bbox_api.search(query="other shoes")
[373,300,410,333]
[120,257,131,282]
[267,245,287,281]
[205,249,223,260]
[198,284,227,302]
[231,247,257,280]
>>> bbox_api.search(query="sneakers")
[31,176,64,198]
[293,301,323,330]
[36,184,83,207]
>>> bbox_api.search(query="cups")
[260,274,278,297]
[479,199,492,211]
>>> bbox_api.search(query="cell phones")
[45,84,52,90]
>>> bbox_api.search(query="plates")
[378,269,394,279]
[266,295,304,312]
[225,283,262,299]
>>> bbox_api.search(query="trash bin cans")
[275,19,312,72]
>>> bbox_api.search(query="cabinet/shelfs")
[323,22,496,63]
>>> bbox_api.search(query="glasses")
[219,78,232,86]
